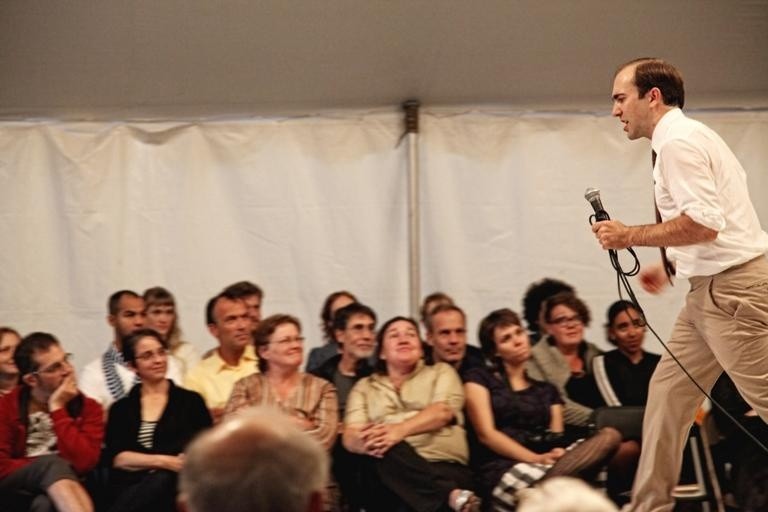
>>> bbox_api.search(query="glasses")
[28,352,72,376]
[135,349,168,359]
[552,315,581,326]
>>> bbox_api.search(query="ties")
[652,148,676,287]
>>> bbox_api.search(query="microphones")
[583,185,618,256]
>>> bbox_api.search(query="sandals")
[454,489,482,512]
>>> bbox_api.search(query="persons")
[464,307,621,512]
[2,331,105,512]
[225,316,339,452]
[0,325,31,394]
[515,279,712,473]
[509,478,620,511]
[78,287,201,403]
[103,329,211,512]
[175,406,331,512]
[182,280,266,404]
[589,56,767,512]
[335,316,481,510]
[304,290,486,420]
